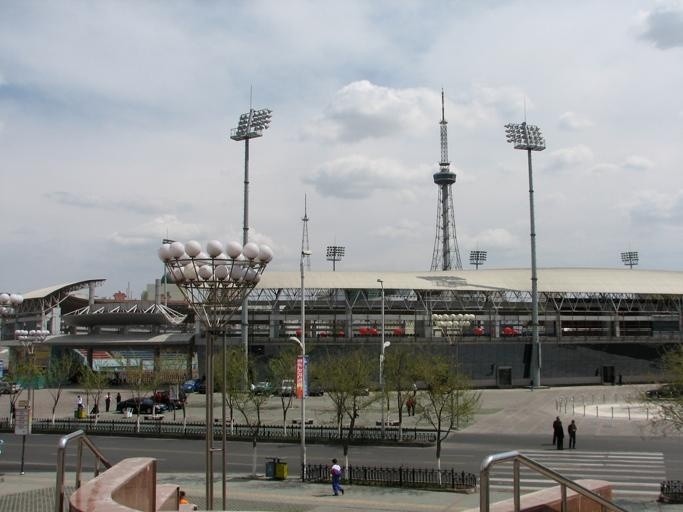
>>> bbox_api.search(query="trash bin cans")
[265,456,288,480]
[74,407,86,418]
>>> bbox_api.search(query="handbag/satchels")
[331,469,342,476]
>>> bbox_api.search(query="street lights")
[621,251,638,269]
[377,278,391,441]
[504,97,546,389]
[0,294,50,474]
[431,314,476,430]
[326,245,345,271]
[289,250,312,481]
[469,250,487,269]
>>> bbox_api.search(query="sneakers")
[333,490,344,496]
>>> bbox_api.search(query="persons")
[90,404,98,414]
[413,383,417,396]
[331,458,344,496]
[78,395,82,408]
[154,389,157,398]
[11,396,16,418]
[167,392,173,412]
[568,420,577,448]
[556,421,564,450]
[179,491,188,504]
[115,392,121,411]
[406,398,414,416]
[155,392,161,403]
[104,392,112,412]
[553,416,562,445]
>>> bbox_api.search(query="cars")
[272,379,324,395]
[116,379,186,415]
